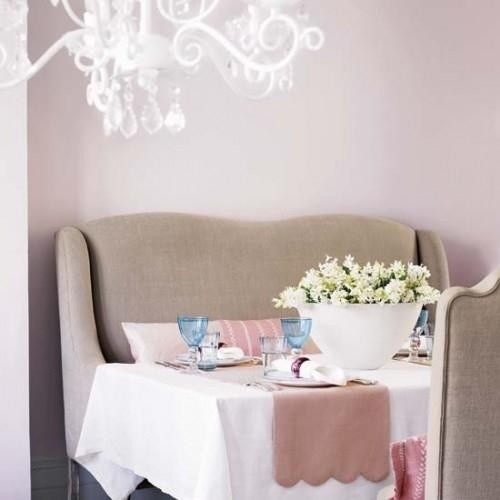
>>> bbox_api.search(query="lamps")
[0,0,323,140]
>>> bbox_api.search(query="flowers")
[271,254,443,309]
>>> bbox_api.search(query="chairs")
[425,268,500,500]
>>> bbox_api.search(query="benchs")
[56,210,451,500]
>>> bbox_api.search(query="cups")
[197,331,220,369]
[260,336,284,376]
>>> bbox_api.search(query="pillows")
[123,316,318,364]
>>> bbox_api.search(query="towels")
[190,346,246,360]
[274,356,348,388]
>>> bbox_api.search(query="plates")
[268,372,347,389]
[193,353,252,366]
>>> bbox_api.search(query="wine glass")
[280,318,312,356]
[176,314,208,376]
[401,310,429,363]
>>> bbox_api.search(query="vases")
[298,304,424,374]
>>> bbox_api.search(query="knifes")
[156,360,186,372]
